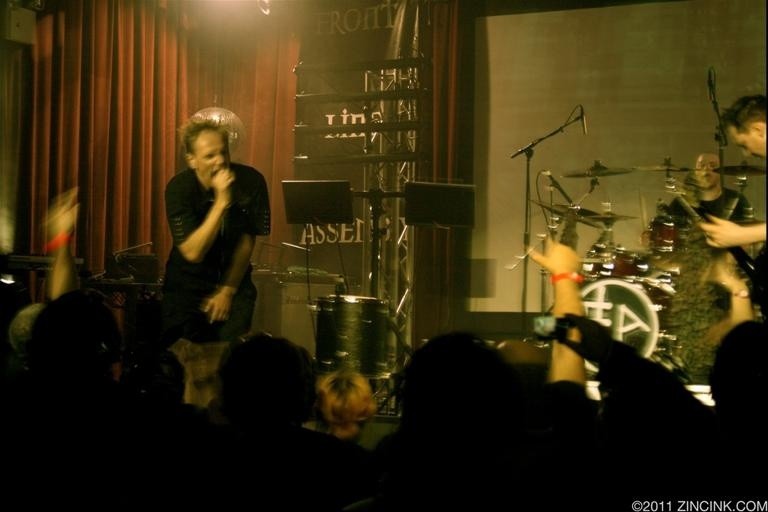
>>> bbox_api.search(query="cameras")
[530,316,570,341]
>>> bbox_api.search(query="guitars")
[665,178,768,324]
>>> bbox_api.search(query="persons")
[0,182,768,509]
[684,151,753,222]
[158,112,273,410]
[696,90,765,324]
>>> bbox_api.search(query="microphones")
[708,70,712,101]
[580,105,588,136]
[549,175,573,204]
[113,241,160,256]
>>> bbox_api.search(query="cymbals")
[528,198,606,230]
[714,164,767,175]
[634,166,694,173]
[551,202,599,218]
[581,212,636,220]
[563,164,636,178]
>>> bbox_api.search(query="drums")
[547,278,664,380]
[316,291,392,383]
[636,274,676,323]
[650,214,684,257]
[581,241,652,287]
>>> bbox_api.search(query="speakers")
[280,180,353,224]
[404,181,476,228]
[315,294,387,375]
